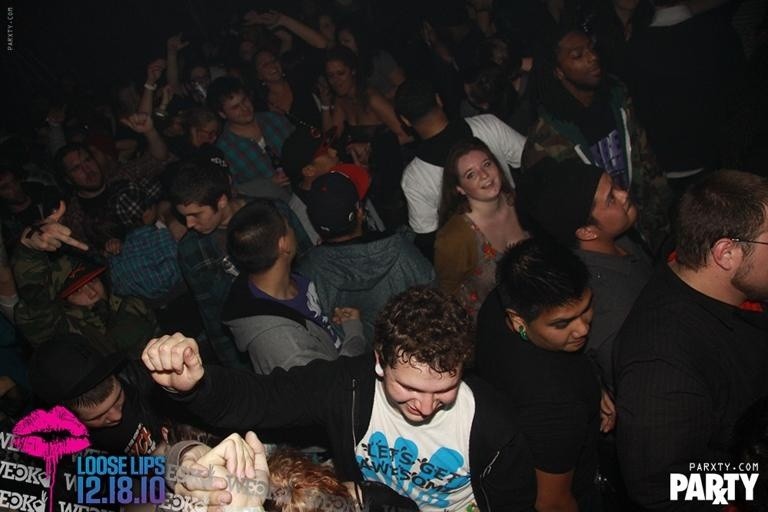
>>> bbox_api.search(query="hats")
[104,175,162,229]
[285,124,339,160]
[44,247,109,298]
[516,156,605,248]
[305,162,370,232]
[27,334,125,403]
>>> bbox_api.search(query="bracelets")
[143,81,157,91]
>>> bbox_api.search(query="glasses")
[725,235,764,248]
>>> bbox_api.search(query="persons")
[386,0,522,109]
[1,173,62,242]
[217,195,368,378]
[139,282,538,511]
[50,111,170,224]
[296,170,435,357]
[389,77,531,233]
[12,205,178,369]
[594,2,623,144]
[166,25,251,105]
[243,10,328,123]
[25,330,167,511]
[277,123,384,234]
[95,56,175,128]
[614,168,768,512]
[144,431,355,512]
[207,75,292,186]
[167,156,316,367]
[527,1,574,49]
[309,9,355,51]
[527,156,653,379]
[519,28,654,243]
[337,24,408,107]
[478,235,615,512]
[92,174,202,338]
[311,48,405,142]
[643,1,768,196]
[173,111,219,160]
[587,0,639,55]
[436,137,531,287]
